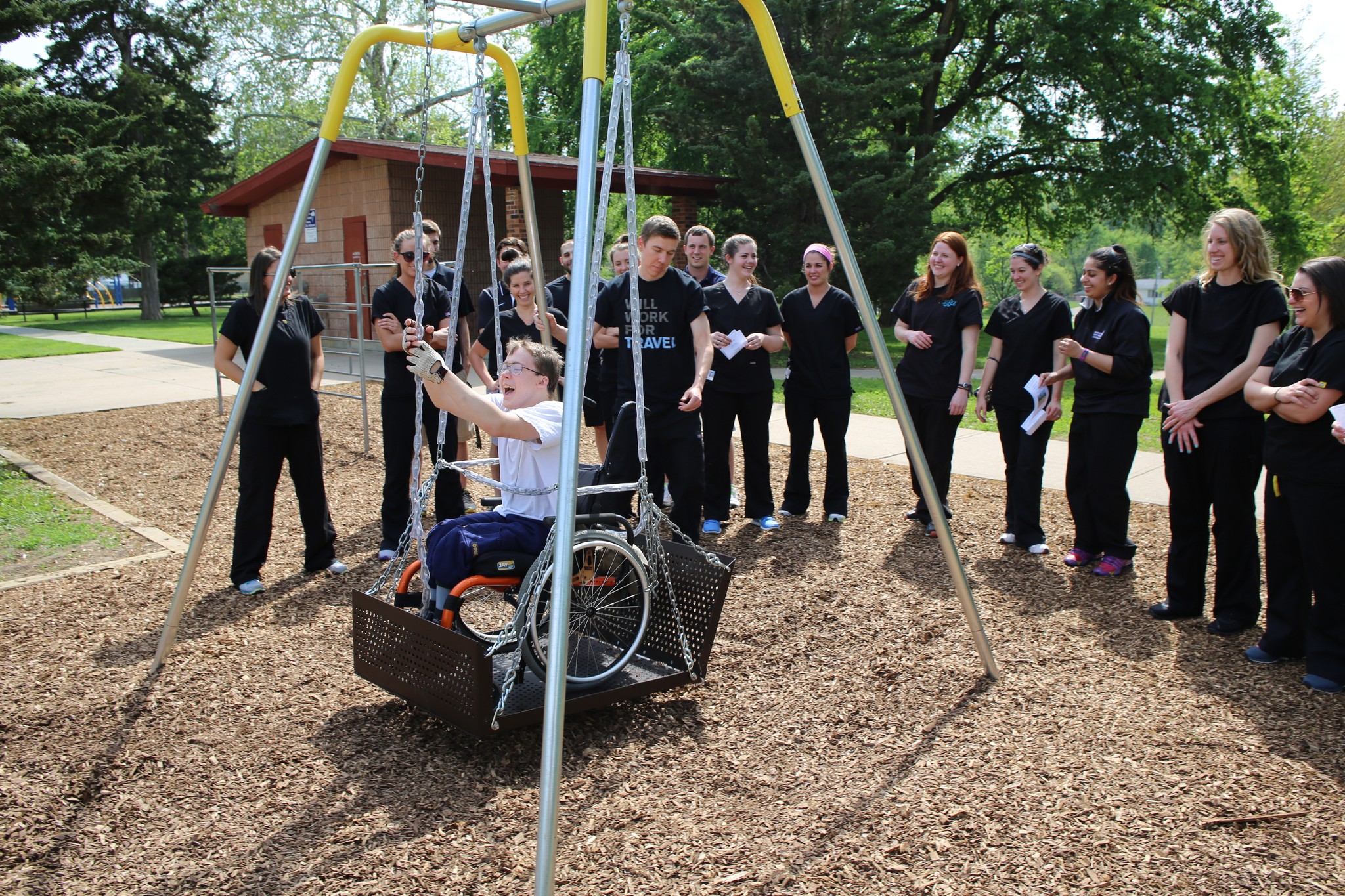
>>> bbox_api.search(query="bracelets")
[258,386,267,391]
[1079,348,1090,362]
[1274,387,1284,402]
[957,387,973,397]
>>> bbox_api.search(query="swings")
[346,0,742,737]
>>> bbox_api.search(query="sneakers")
[662,482,671,506]
[461,487,478,512]
[1093,555,1134,576]
[751,515,781,530]
[904,507,920,520]
[702,520,722,534]
[1029,544,1050,554]
[828,514,846,522]
[1302,675,1343,693]
[1243,644,1307,663]
[730,484,742,508]
[777,508,792,516]
[1063,548,1105,566]
[998,533,1016,543]
[410,490,428,516]
[925,521,937,537]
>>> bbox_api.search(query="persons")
[974,242,1074,554]
[701,234,785,535]
[1147,208,1290,637]
[777,243,865,522]
[371,219,642,635]
[533,215,714,547]
[890,231,984,538]
[1243,257,1345,694]
[663,225,742,508]
[214,246,349,595]
[1039,243,1154,576]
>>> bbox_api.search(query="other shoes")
[324,560,347,574]
[378,549,396,560]
[239,580,266,595]
[1206,619,1257,636]
[1148,599,1203,619]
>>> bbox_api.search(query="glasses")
[265,268,297,279]
[1285,287,1323,303]
[1013,242,1039,254]
[399,251,430,262]
[500,250,510,263]
[497,362,544,376]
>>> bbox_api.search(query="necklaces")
[725,283,749,296]
[1022,300,1038,312]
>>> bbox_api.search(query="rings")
[756,343,758,347]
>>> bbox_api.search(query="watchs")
[957,383,972,388]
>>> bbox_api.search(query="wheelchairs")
[398,394,657,695]
